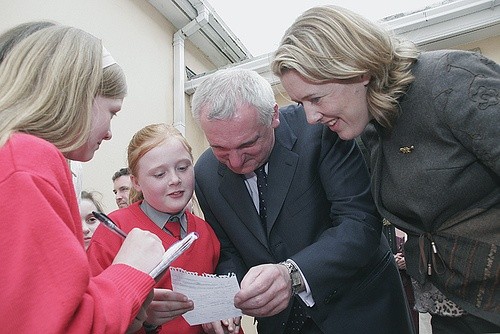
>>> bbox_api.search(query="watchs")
[280,261,304,295]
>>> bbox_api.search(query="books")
[149,232,199,280]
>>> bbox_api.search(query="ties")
[254,165,306,333]
[164,222,182,241]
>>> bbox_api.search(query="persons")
[382,219,419,334]
[0,20,165,334]
[87,121,245,334]
[271,4,500,334]
[191,68,413,334]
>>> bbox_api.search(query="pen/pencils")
[92,211,127,238]
